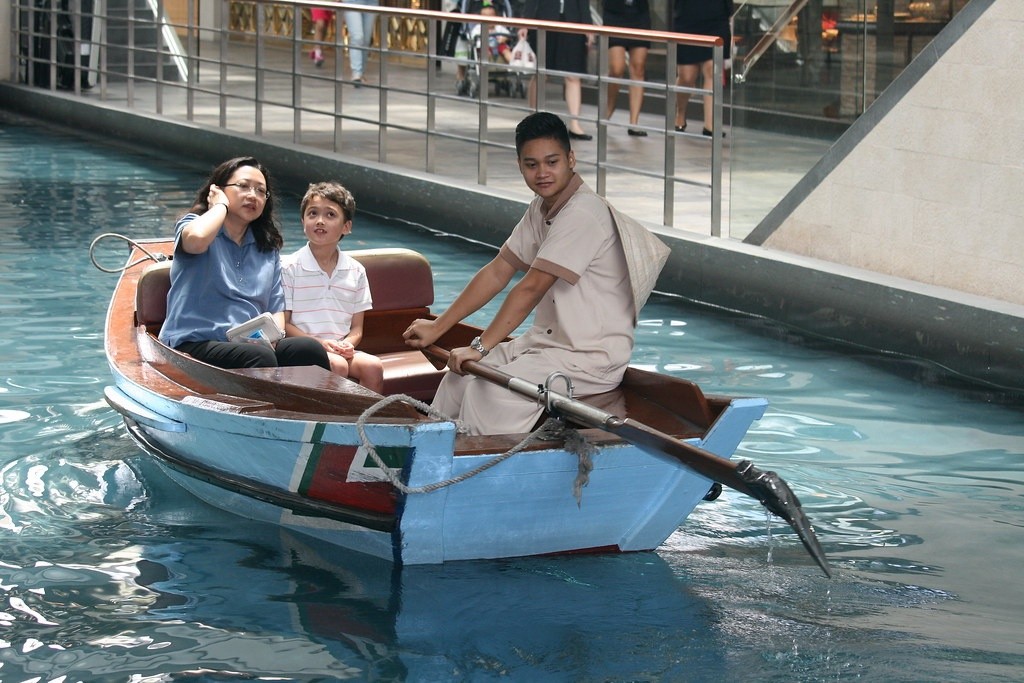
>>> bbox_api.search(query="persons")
[157,156,333,374]
[277,182,384,394]
[310,0,734,141]
[402,112,634,436]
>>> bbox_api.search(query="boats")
[101,236,767,566]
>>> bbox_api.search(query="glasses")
[221,182,270,199]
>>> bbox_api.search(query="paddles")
[406,332,833,581]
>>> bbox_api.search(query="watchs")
[471,336,490,356]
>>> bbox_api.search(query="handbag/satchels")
[507,39,538,76]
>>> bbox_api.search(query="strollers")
[456,0,532,100]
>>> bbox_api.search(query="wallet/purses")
[226,312,286,344]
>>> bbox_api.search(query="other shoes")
[628,129,647,136]
[675,122,688,132]
[570,129,592,141]
[702,127,726,138]
[314,55,323,67]
[350,73,364,88]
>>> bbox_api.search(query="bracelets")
[212,203,229,213]
